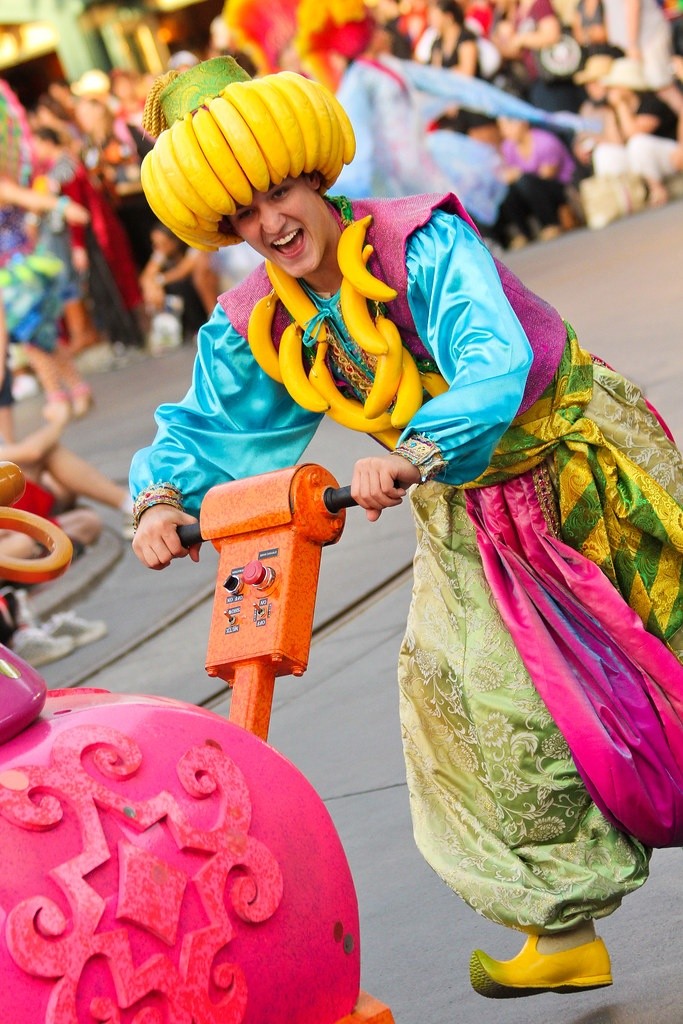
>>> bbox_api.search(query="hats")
[141,55,354,255]
[607,58,649,92]
[574,56,614,86]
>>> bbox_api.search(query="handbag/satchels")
[577,176,648,226]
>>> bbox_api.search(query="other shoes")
[13,633,75,667]
[534,227,559,246]
[471,923,616,999]
[42,613,104,647]
[506,234,528,256]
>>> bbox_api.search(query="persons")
[0,0,683,429]
[0,300,130,667]
[129,56,683,999]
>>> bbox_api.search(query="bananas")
[140,71,355,252]
[245,215,449,451]
